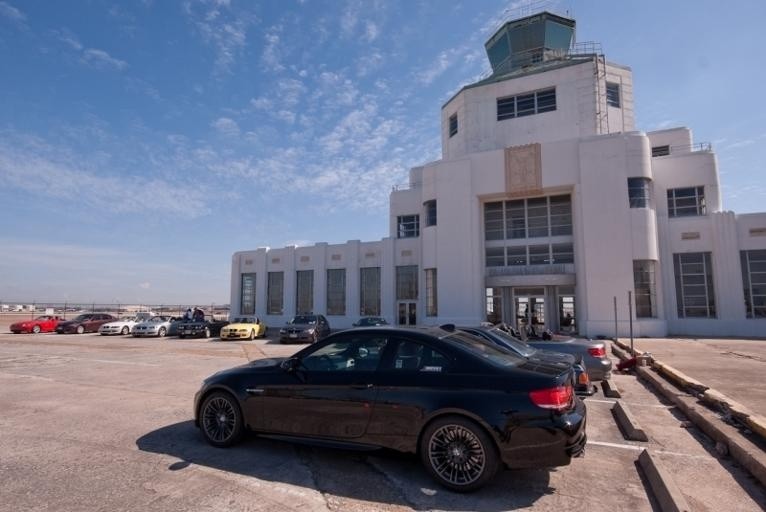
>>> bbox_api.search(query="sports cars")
[488,322,612,382]
[130,315,192,337]
[220,315,268,341]
[177,314,229,337]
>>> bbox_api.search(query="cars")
[360,326,597,407]
[98,315,147,335]
[9,315,66,333]
[279,314,330,344]
[54,313,117,334]
[193,322,588,493]
[352,317,389,326]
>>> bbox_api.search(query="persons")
[184,307,193,320]
[194,308,206,322]
[559,305,572,327]
